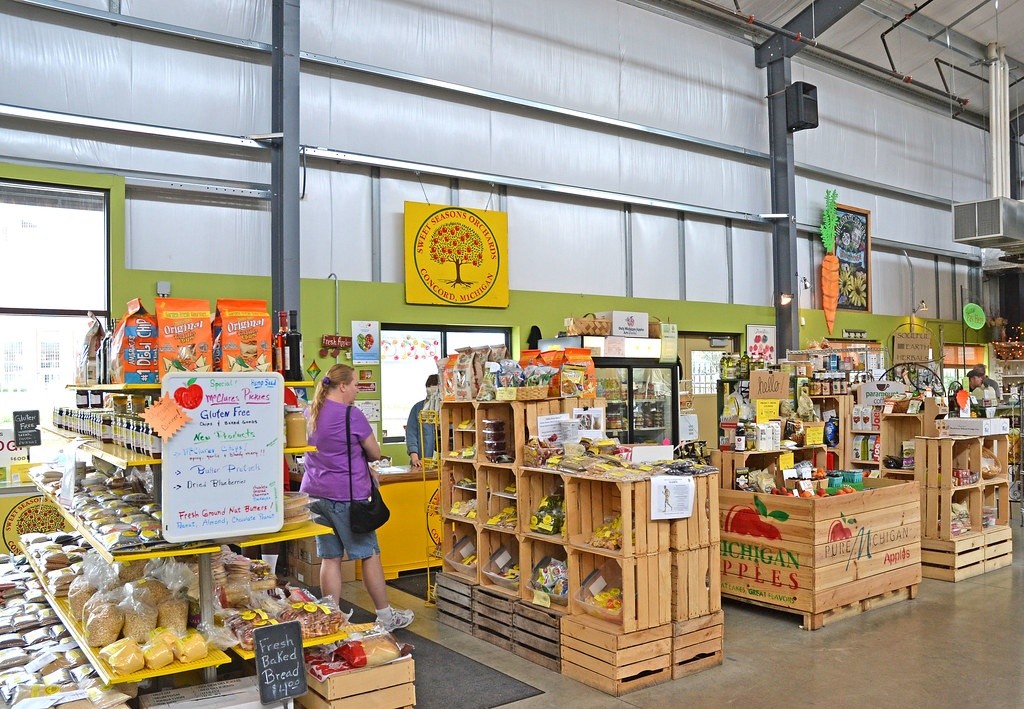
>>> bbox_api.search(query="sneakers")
[374,607,415,634]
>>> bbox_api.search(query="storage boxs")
[526,575,569,606]
[296,658,416,709]
[8,463,42,483]
[943,419,1009,436]
[139,674,294,709]
[537,311,682,364]
[444,531,477,578]
[573,560,623,627]
[482,538,519,592]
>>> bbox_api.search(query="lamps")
[771,288,791,308]
[795,272,812,289]
[916,300,928,312]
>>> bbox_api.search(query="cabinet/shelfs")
[17,358,1024,709]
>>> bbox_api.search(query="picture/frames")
[834,203,872,314]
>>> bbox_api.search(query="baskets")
[566,313,612,336]
[981,446,1002,479]
[884,398,921,413]
[495,384,549,401]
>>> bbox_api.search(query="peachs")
[812,467,827,477]
[771,487,858,499]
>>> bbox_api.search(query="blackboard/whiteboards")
[162,371,284,543]
[253,621,308,705]
[13,410,42,446]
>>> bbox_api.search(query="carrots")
[821,252,839,335]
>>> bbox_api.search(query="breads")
[186,551,402,668]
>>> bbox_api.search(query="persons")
[241,387,297,586]
[967,364,1003,403]
[662,486,672,511]
[407,374,453,466]
[297,363,414,632]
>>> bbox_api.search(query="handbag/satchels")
[348,485,391,534]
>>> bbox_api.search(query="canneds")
[53,389,162,460]
[284,404,309,448]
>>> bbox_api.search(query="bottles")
[734,423,745,452]
[606,414,621,428]
[809,371,847,396]
[272,311,304,380]
[719,351,764,379]
[597,378,620,400]
